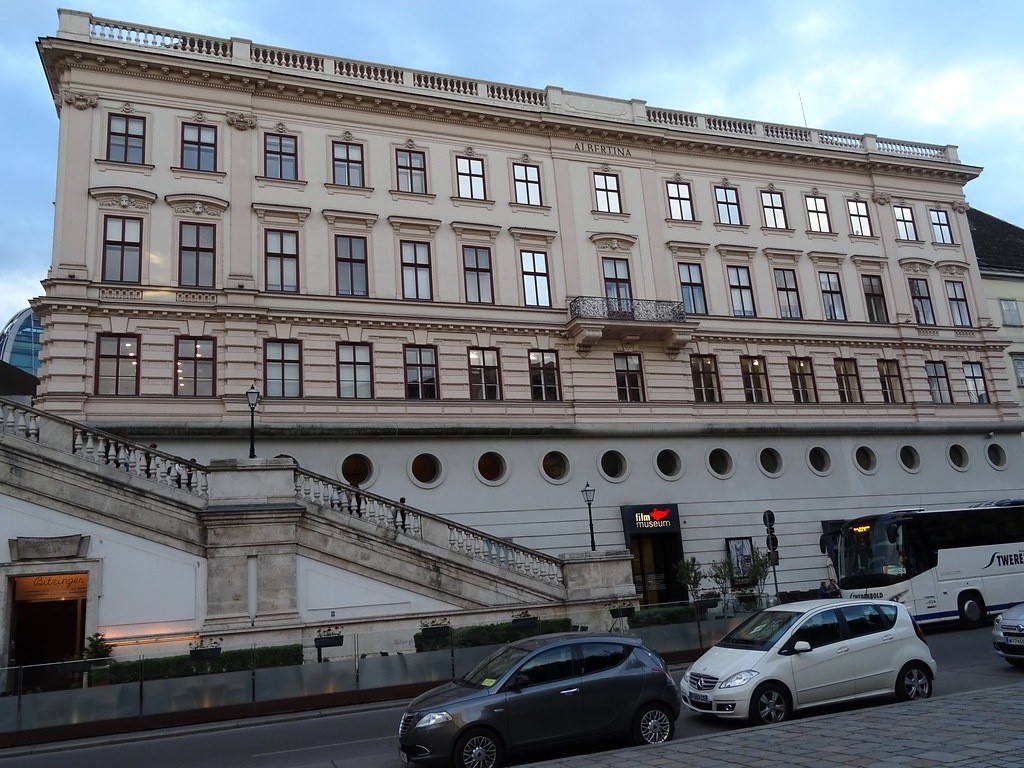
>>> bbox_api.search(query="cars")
[398,631,680,768]
[680,599,938,726]
[991,603,1024,666]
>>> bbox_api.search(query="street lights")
[245,383,260,459]
[579,481,597,553]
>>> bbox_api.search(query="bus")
[820,499,1024,629]
